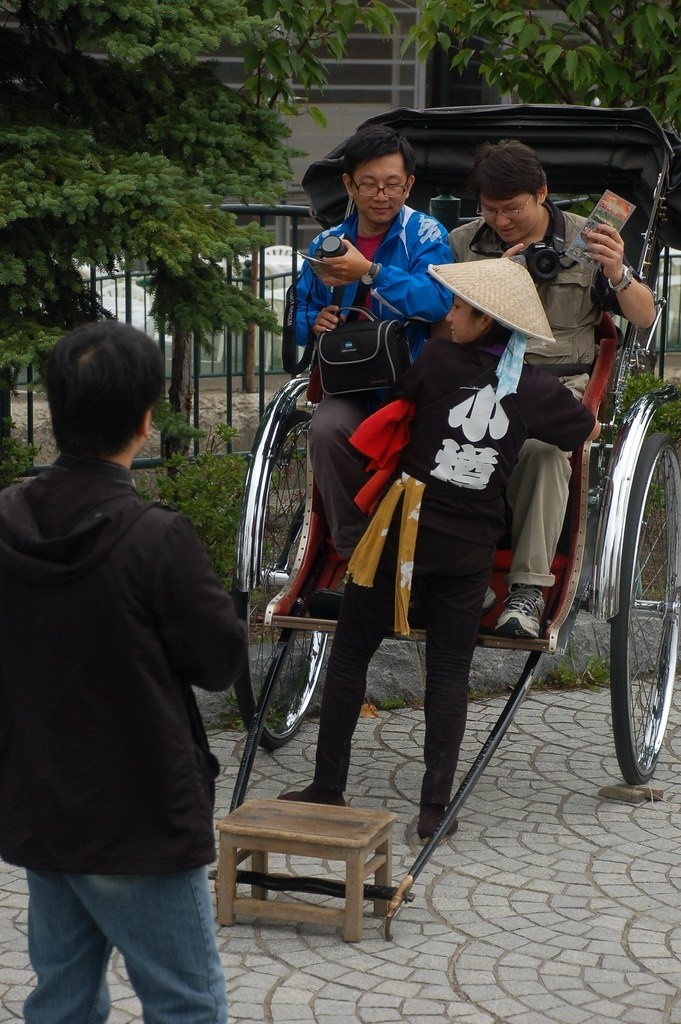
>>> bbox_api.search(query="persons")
[282,125,460,619]
[0,321,246,1024]
[275,256,601,851]
[421,141,655,640]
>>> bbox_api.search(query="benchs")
[265,305,620,655]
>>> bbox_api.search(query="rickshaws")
[203,104,681,945]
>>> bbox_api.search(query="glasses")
[476,191,531,219]
[351,174,409,197]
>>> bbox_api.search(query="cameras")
[517,242,561,283]
[322,235,347,258]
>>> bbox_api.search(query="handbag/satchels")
[315,306,414,396]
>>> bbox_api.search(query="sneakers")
[482,586,498,617]
[493,583,545,639]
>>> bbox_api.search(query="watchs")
[608,264,632,292]
[361,262,377,285]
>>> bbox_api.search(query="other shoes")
[417,815,458,846]
[308,567,347,618]
[277,784,346,806]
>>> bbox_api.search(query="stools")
[215,798,399,943]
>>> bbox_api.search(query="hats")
[427,255,557,345]
